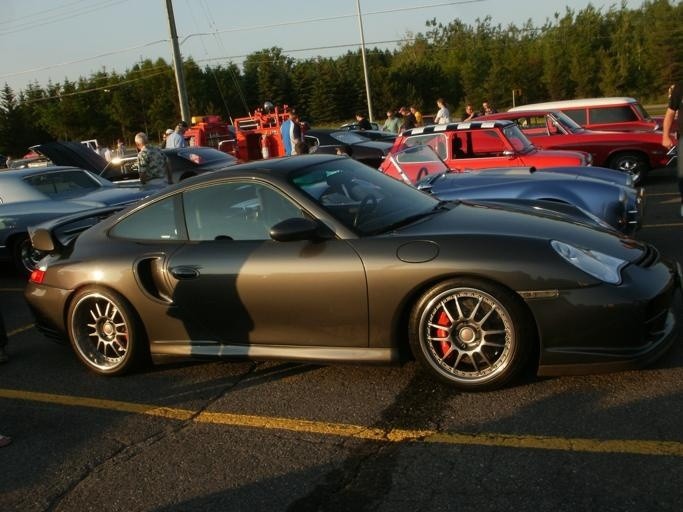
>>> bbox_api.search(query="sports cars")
[20,154,683,392]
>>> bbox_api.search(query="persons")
[134,131,173,187]
[5,156,12,169]
[354,98,498,136]
[161,134,166,149]
[165,129,186,148]
[336,146,350,158]
[96,139,126,163]
[280,109,302,156]
[662,80,683,218]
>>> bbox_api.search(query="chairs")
[257,186,291,225]
[195,198,244,240]
[453,137,465,158]
